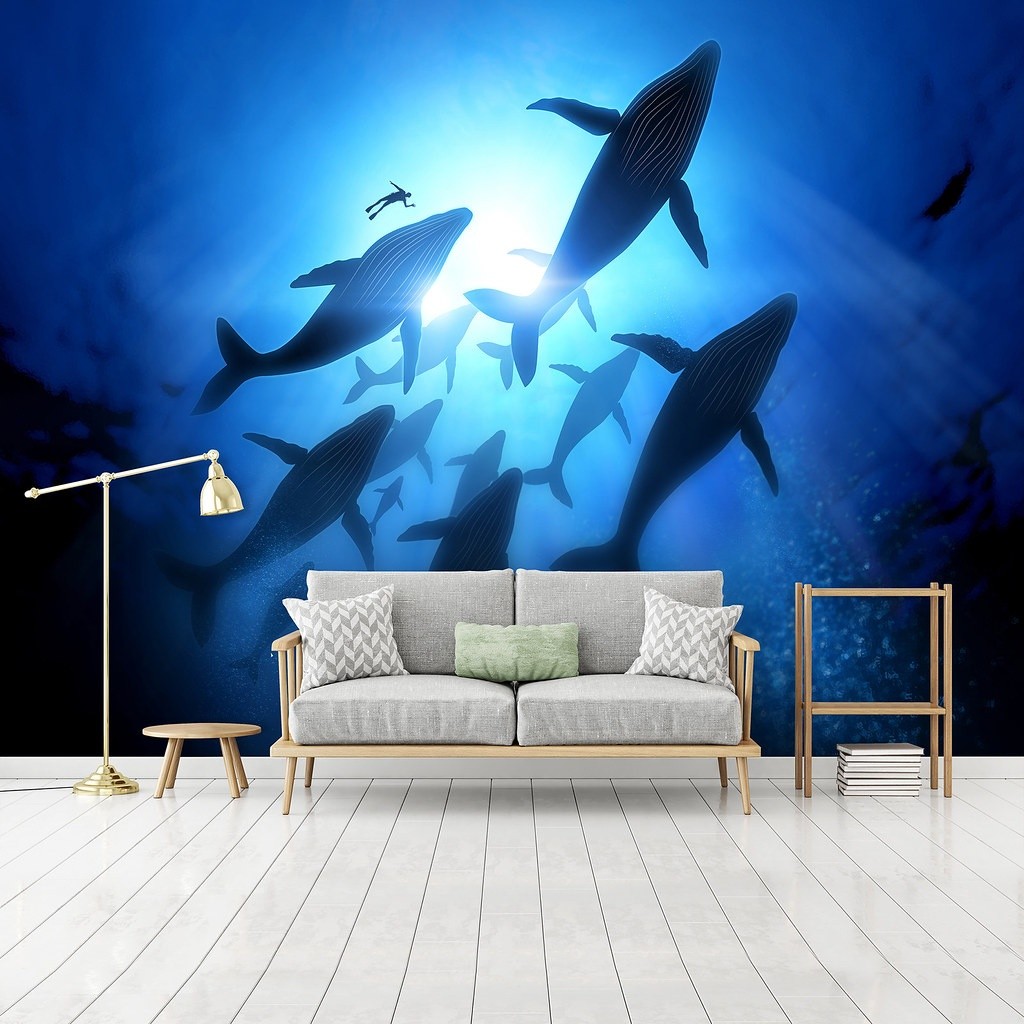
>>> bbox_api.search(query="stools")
[143,723,261,799]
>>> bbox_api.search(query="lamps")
[24,448,245,796]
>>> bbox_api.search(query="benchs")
[269,568,761,815]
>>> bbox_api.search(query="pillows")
[454,621,579,681]
[281,582,410,695]
[625,584,744,692]
